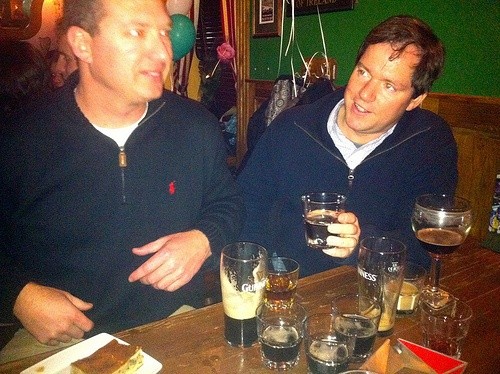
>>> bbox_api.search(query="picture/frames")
[252,1,283,37]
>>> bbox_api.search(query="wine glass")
[412,193,471,308]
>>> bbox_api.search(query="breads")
[70,339,144,374]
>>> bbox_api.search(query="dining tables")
[0,226,500,374]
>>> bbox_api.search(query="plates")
[20,333,162,374]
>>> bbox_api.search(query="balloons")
[165,0,192,17]
[166,11,197,65]
[22,0,32,16]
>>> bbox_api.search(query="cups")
[304,313,357,374]
[396,261,427,318]
[357,237,406,337]
[302,193,347,248]
[331,294,382,363]
[267,257,300,312]
[421,298,472,359]
[256,301,307,370]
[339,370,378,374]
[220,241,268,348]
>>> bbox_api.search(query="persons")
[0,40,53,115]
[234,14,460,285]
[0,0,79,97]
[1,0,243,367]
